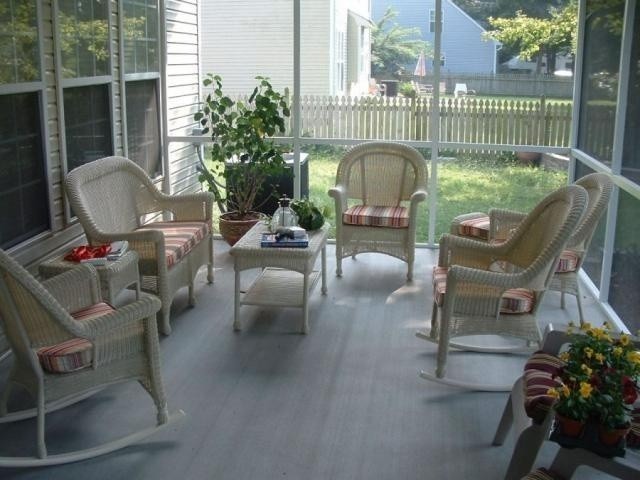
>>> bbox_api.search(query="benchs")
[65,155,215,336]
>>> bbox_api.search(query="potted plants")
[290,195,331,229]
[194,72,295,248]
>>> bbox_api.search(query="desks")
[227,219,330,337]
[38,249,141,310]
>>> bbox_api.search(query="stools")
[450,210,491,242]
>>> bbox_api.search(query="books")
[260,232,308,247]
[64,240,129,265]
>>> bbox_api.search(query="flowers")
[547,320,640,424]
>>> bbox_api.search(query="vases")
[557,408,587,438]
[597,417,632,444]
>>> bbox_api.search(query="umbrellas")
[411,49,426,83]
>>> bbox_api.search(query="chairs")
[440,81,447,96]
[489,334,640,479]
[327,140,429,283]
[455,84,467,97]
[414,183,590,393]
[0,247,170,469]
[450,171,614,335]
[411,80,433,98]
[516,445,640,479]
